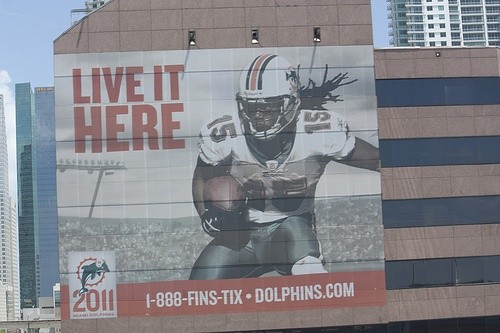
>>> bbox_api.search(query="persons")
[190,55,379,279]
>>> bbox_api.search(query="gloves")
[201,205,251,243]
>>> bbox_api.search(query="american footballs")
[204,176,246,219]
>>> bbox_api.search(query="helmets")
[235,52,300,143]
